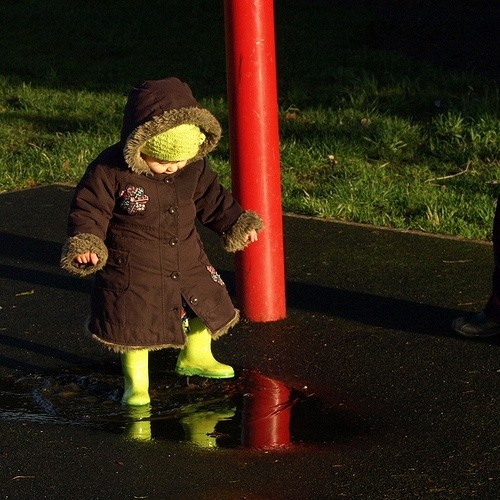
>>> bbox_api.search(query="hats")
[138,124,206,160]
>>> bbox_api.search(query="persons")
[59,77,262,405]
[123,409,235,449]
[452,193,500,338]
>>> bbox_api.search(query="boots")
[120,347,151,406]
[173,319,235,379]
